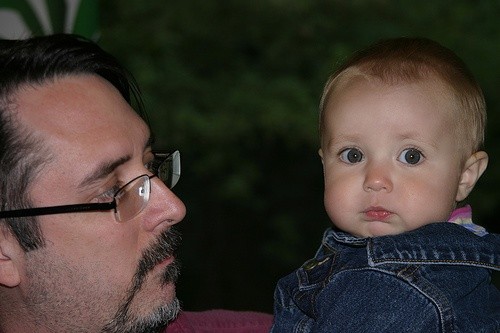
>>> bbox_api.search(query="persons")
[268,38,500,333]
[0,33,274,333]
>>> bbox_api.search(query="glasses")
[0,150,180,223]
